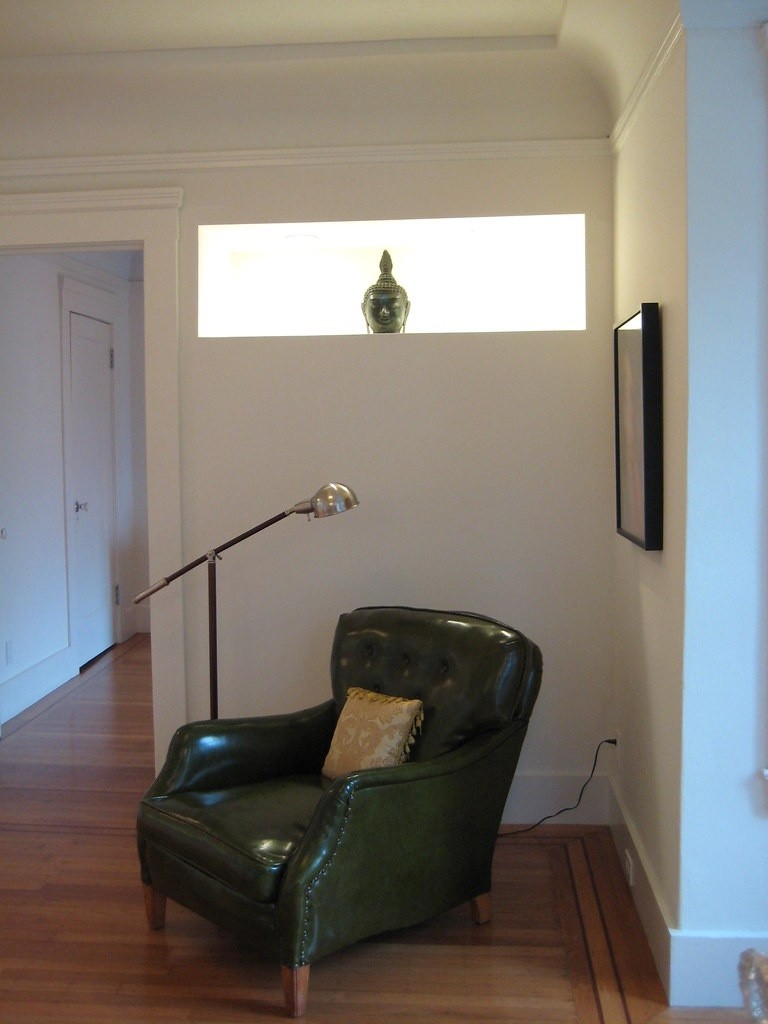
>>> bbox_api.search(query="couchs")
[135,604,545,1017]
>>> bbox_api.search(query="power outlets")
[622,849,634,886]
[613,729,622,766]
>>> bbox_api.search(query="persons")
[361,250,410,334]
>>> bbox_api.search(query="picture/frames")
[612,302,666,552]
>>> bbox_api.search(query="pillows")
[321,688,426,785]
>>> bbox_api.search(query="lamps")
[134,483,360,719]
